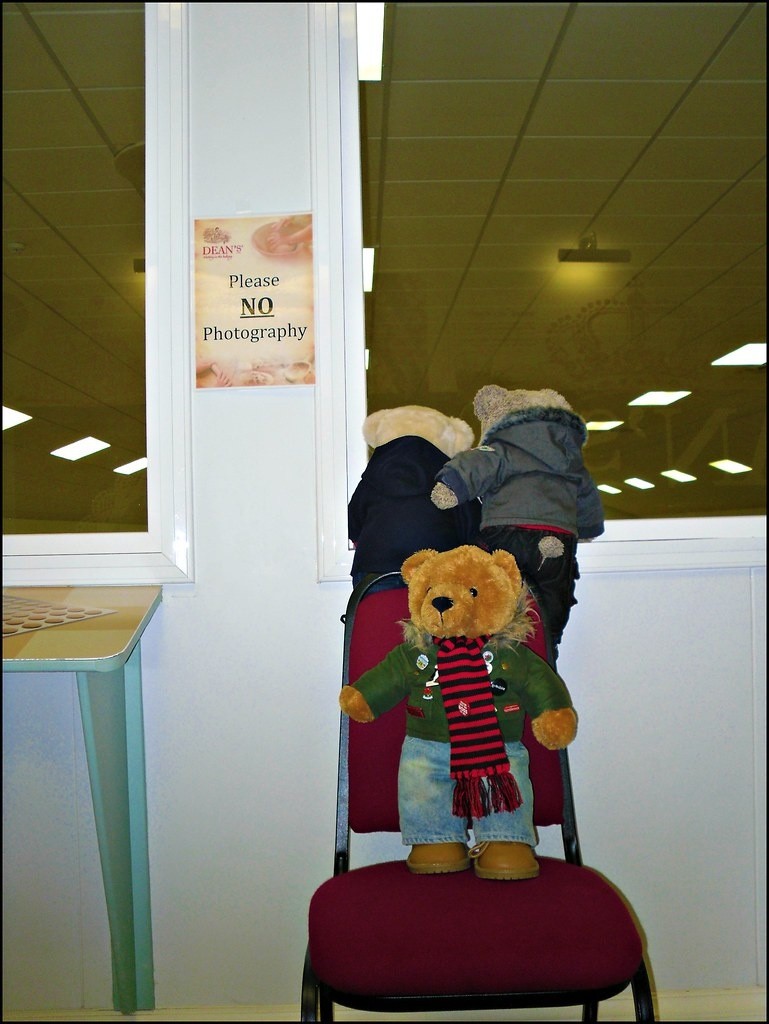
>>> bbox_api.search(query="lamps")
[558,232,631,263]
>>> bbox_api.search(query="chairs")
[301,571,656,1024]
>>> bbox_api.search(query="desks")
[3,586,163,1018]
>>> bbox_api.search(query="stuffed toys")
[430,384,605,661]
[340,545,578,880]
[348,406,483,596]
